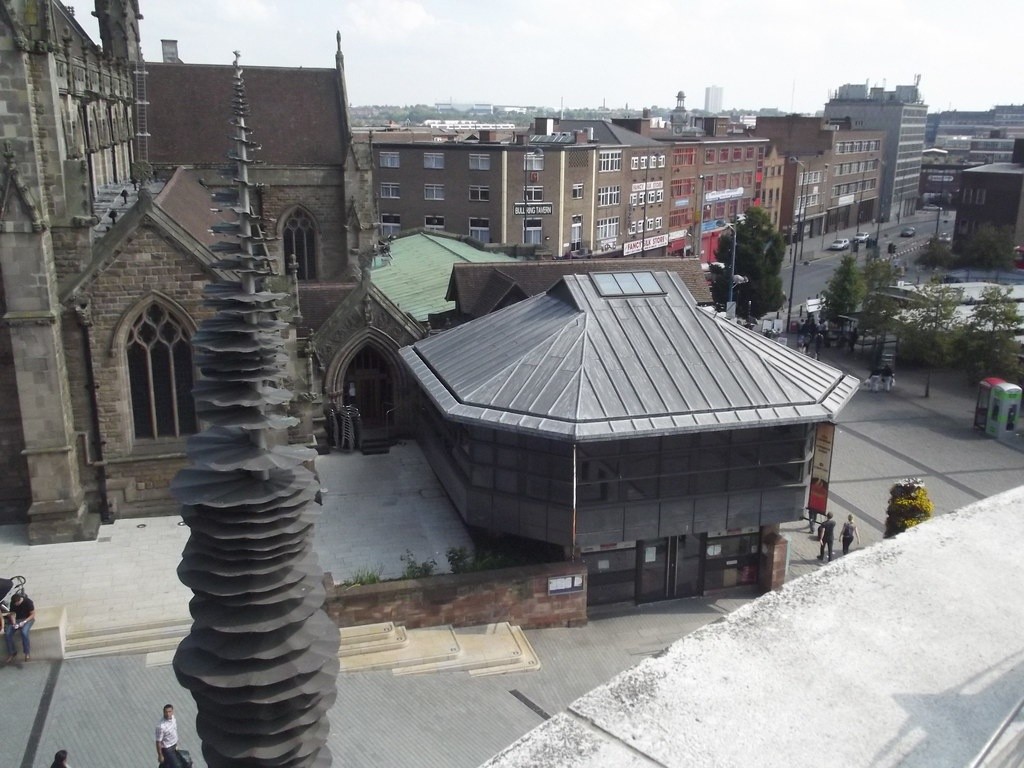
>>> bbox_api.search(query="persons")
[797,314,895,393]
[155,704,185,768]
[0,593,35,663]
[804,501,861,563]
[51,749,71,768]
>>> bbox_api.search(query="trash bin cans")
[790,321,798,334]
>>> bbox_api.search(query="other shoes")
[6,651,17,663]
[25,653,30,661]
[817,556,823,559]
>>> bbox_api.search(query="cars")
[854,232,869,242]
[901,227,917,236]
[923,203,943,211]
[830,238,849,251]
[931,273,962,286]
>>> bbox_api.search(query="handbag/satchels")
[864,378,870,385]
[159,750,193,768]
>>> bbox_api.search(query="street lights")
[787,157,807,334]
[523,142,543,243]
[717,220,736,302]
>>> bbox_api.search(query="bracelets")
[24,620,26,623]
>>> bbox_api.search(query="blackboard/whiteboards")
[762,319,783,333]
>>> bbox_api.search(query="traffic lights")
[888,243,896,254]
